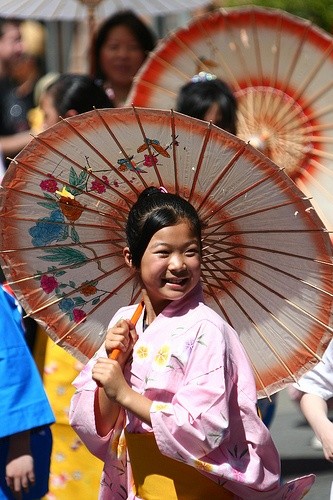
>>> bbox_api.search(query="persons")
[68,186,282,500]
[63,8,158,118]
[0,284,36,500]
[0,13,47,156]
[38,70,115,134]
[299,333,333,462]
[173,72,239,141]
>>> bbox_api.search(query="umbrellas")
[120,5,333,235]
[0,103,333,404]
